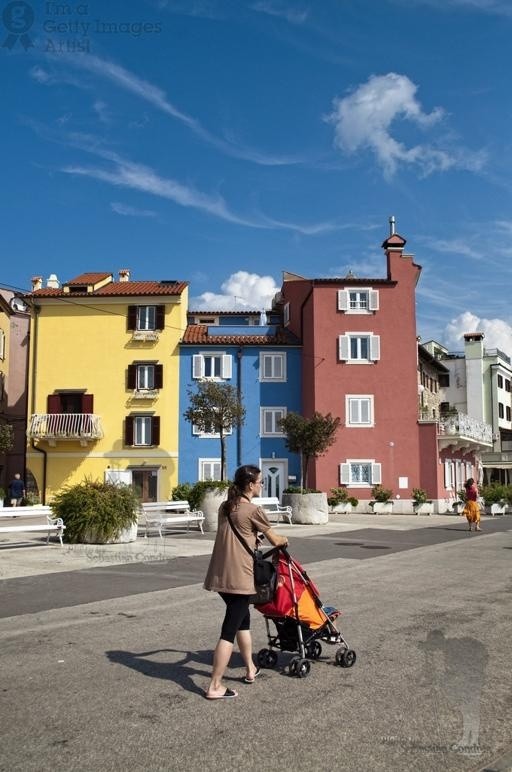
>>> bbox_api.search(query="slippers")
[205,687,240,700]
[244,665,261,683]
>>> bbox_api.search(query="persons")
[462,478,483,531]
[6,474,25,518]
[201,464,291,700]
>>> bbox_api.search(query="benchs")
[0,505,66,547]
[141,500,205,539]
[250,496,292,526]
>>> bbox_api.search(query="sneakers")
[467,527,483,531]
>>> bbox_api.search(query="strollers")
[253,534,356,678]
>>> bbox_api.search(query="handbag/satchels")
[248,561,276,605]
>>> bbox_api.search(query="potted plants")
[48,473,143,544]
[327,487,358,514]
[368,484,394,515]
[188,480,235,533]
[282,488,328,525]
[451,480,512,517]
[412,488,434,516]
